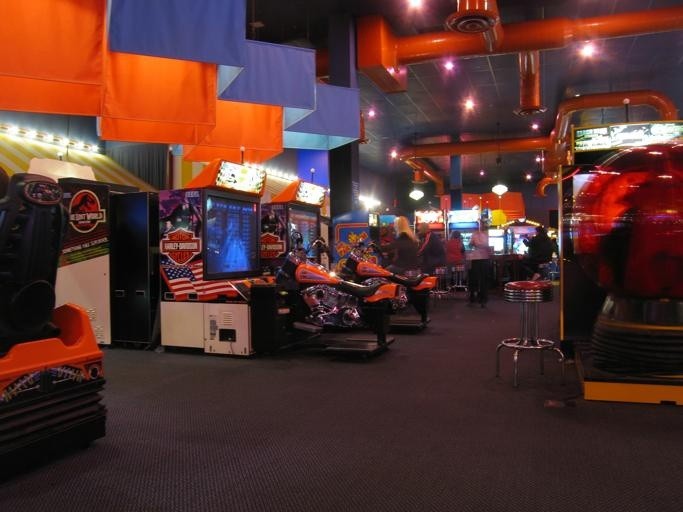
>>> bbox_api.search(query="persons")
[313,237,334,271]
[468,220,492,306]
[445,230,465,264]
[294,237,306,264]
[513,235,529,255]
[416,223,447,275]
[345,242,366,271]
[373,216,424,279]
[521,227,555,281]
[283,247,304,281]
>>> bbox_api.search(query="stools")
[494,280,564,388]
[451,264,469,296]
[432,266,449,301]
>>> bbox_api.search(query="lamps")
[491,157,508,198]
[409,185,425,200]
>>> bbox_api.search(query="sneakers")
[531,272,540,280]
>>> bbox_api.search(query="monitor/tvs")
[285,203,321,260]
[200,188,262,281]
[566,167,610,244]
[512,234,538,254]
[449,228,479,251]
[488,229,505,255]
